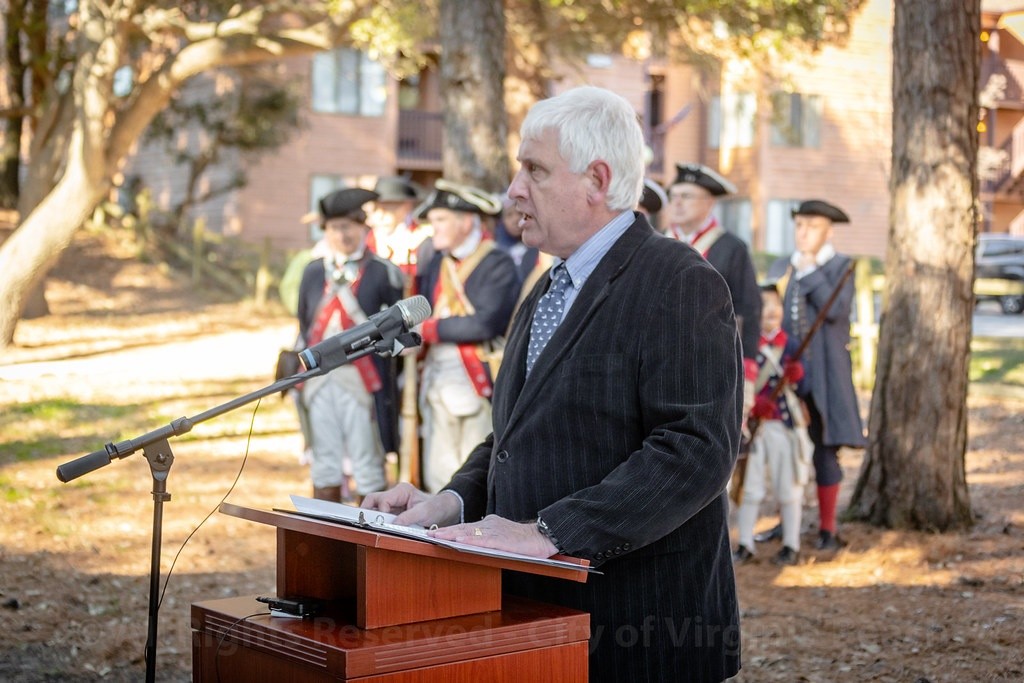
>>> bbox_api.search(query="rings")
[475,527,482,537]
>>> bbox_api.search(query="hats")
[673,163,738,197]
[320,188,379,217]
[792,200,850,224]
[418,178,501,220]
[639,179,670,212]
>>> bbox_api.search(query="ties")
[525,268,571,378]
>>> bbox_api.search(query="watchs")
[537,516,565,555]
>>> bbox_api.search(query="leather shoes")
[733,524,838,566]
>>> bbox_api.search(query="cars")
[974,233,1024,313]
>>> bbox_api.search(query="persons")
[361,86,742,683]
[298,163,868,563]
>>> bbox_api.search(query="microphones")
[298,294,431,371]
